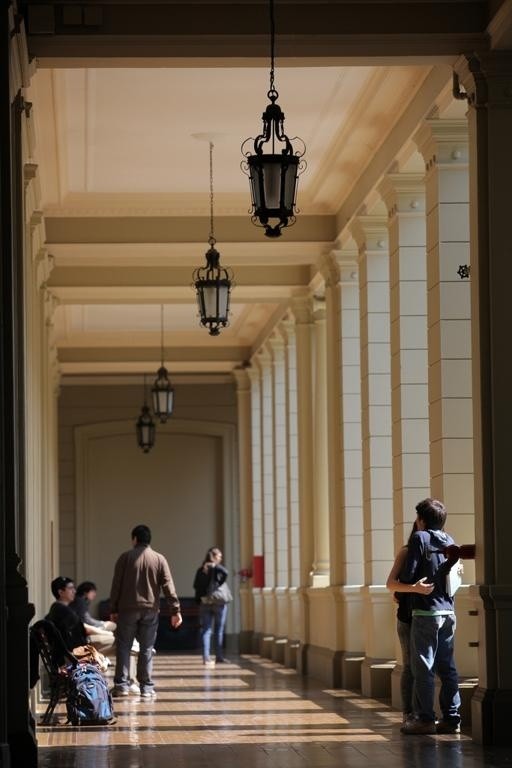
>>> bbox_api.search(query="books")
[445,557,462,598]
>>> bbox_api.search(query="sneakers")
[141,689,157,697]
[128,684,141,695]
[204,659,229,665]
[109,683,129,697]
[400,713,457,734]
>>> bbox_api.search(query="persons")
[194,547,231,665]
[110,525,182,697]
[50,575,113,673]
[386,518,465,722]
[393,499,462,735]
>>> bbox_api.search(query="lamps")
[237,0,308,238]
[188,140,237,337]
[136,373,156,454]
[150,303,173,423]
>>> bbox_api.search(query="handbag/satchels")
[58,660,118,726]
[73,645,108,673]
[201,582,234,605]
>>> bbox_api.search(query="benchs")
[31,619,81,726]
